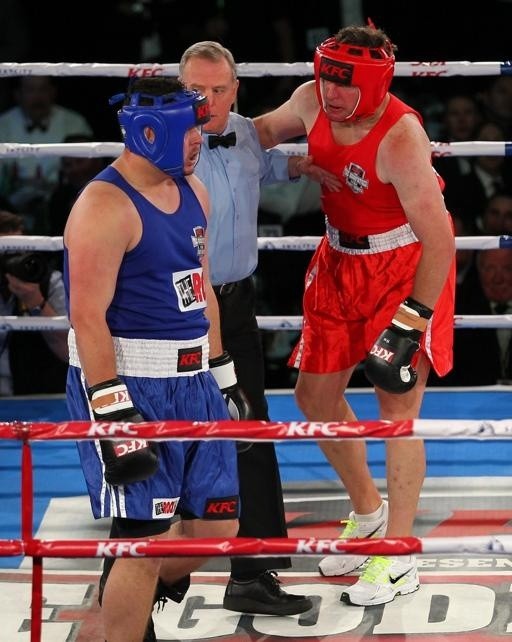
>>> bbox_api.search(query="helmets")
[314,36,396,125]
[108,89,210,179]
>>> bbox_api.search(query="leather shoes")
[93,573,191,642]
[223,574,313,615]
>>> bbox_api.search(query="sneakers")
[318,500,421,606]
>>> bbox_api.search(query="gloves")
[208,350,257,453]
[364,297,433,394]
[85,377,162,486]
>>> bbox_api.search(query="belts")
[216,277,257,297]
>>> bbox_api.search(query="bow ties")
[207,130,237,150]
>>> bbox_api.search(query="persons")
[59,75,252,642]
[99,40,343,617]
[430,74,512,387]
[254,25,460,606]
[0,75,102,395]
